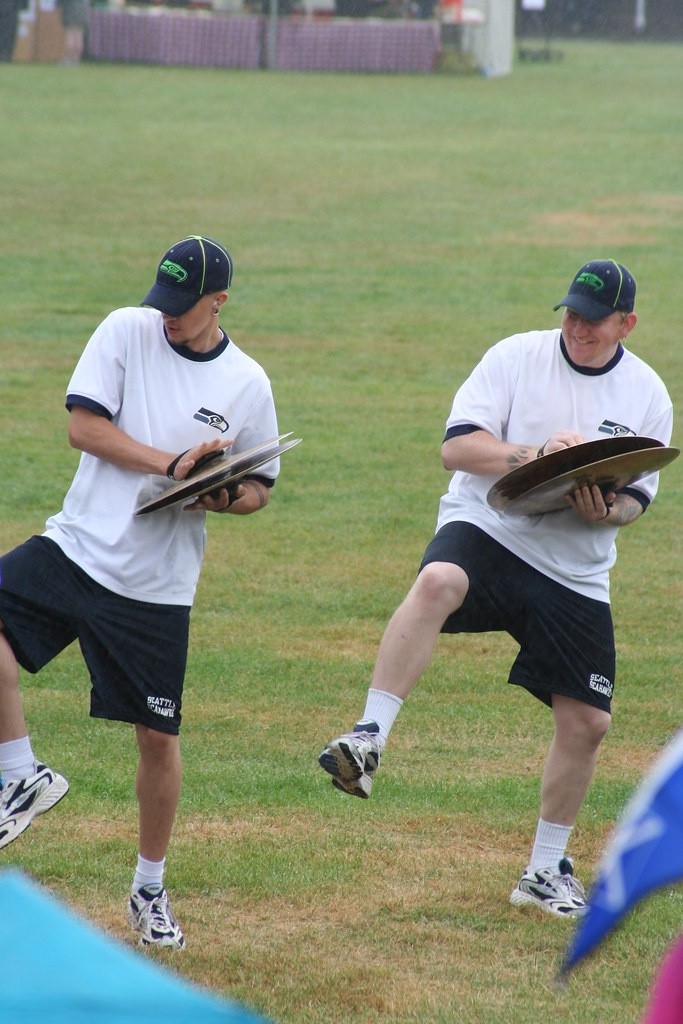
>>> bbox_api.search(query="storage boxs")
[11,10,66,63]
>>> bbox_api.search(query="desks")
[272,17,445,72]
[82,7,262,70]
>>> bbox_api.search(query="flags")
[552,731,683,980]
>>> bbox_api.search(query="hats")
[139,235,233,318]
[553,258,636,321]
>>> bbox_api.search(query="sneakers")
[127,882,185,952]
[509,857,590,919]
[0,759,69,850]
[318,718,387,799]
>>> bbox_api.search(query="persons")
[0,235,280,954]
[318,259,673,921]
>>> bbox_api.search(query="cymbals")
[488,438,680,519]
[135,431,304,523]
